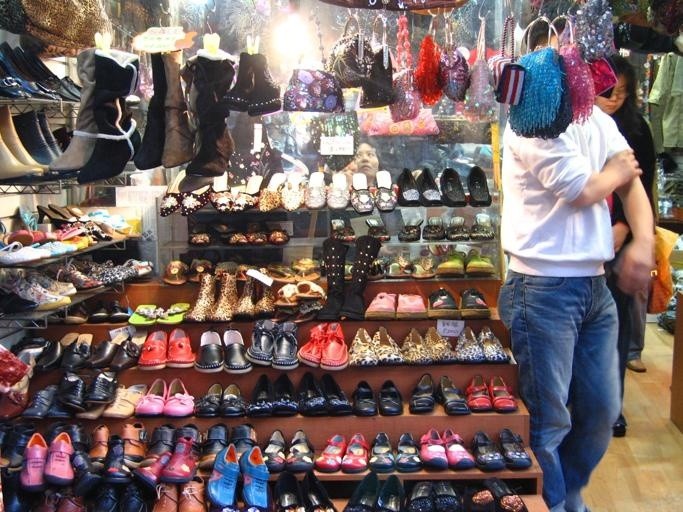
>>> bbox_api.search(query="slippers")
[329,212,496,243]
[158,300,189,327]
[1,200,141,264]
[128,302,163,328]
[274,277,322,323]
[369,251,434,279]
[162,254,322,283]
[160,170,396,217]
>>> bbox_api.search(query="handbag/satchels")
[281,0,620,141]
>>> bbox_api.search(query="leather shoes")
[2,46,83,100]
[613,421,626,437]
[626,358,647,372]
[0,321,533,512]
[397,167,492,207]
[428,286,460,320]
[0,286,36,311]
[185,217,290,245]
[459,287,492,321]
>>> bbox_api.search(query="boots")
[0,105,76,179]
[224,50,252,113]
[51,48,233,186]
[316,237,348,320]
[341,234,381,323]
[186,268,274,323]
[248,52,281,116]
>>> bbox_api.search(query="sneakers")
[108,298,130,322]
[467,243,494,277]
[366,290,398,319]
[7,258,151,308]
[437,248,466,277]
[50,302,89,325]
[397,290,427,320]
[92,300,107,322]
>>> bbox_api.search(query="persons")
[588,52,654,438]
[495,27,654,512]
[624,65,659,374]
[339,143,380,186]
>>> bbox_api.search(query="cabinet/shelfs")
[0,95,544,511]
